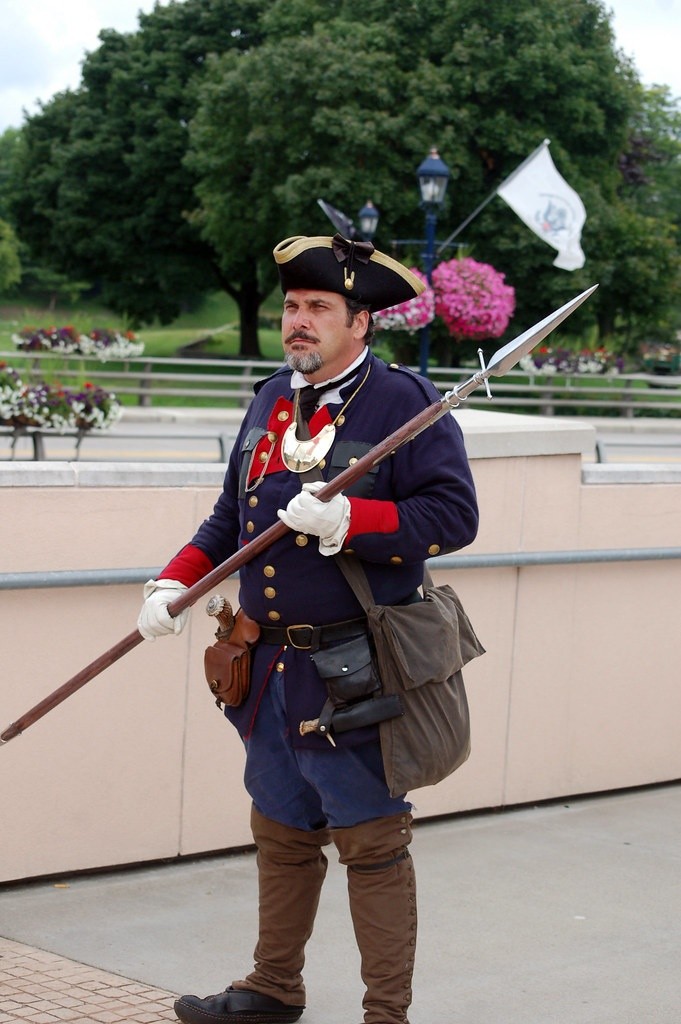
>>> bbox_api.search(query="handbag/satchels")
[367,584,487,798]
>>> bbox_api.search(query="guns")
[204,590,235,634]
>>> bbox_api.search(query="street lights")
[411,148,450,381]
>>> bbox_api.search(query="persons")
[134,232,489,1024]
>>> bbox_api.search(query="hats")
[273,234,427,314]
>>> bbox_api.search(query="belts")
[257,616,369,643]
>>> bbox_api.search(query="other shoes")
[174,985,304,1024]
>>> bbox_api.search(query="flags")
[493,134,590,273]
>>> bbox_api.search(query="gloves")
[136,579,189,643]
[278,480,352,557]
